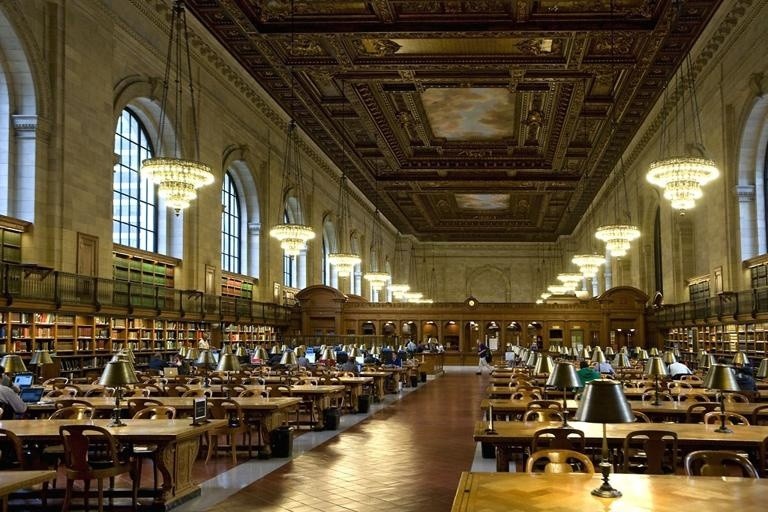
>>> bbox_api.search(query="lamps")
[364,208,433,303]
[268,119,316,259]
[141,1,215,217]
[644,42,725,215]
[327,172,362,280]
[536,122,641,304]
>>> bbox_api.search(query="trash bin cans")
[358,395,370,413]
[411,376,418,387]
[421,372,426,382]
[273,426,293,457]
[481,441,496,459]
[325,406,340,430]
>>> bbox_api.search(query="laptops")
[163,367,179,378]
[212,353,219,364]
[355,364,361,373]
[19,388,45,405]
[305,352,316,365]
[13,373,34,388]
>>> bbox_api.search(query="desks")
[0,419,230,510]
[454,473,768,510]
[472,353,766,462]
[2,469,56,509]
[1,336,437,464]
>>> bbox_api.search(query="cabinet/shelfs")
[656,313,768,376]
[0,299,290,361]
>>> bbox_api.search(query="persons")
[0,366,27,414]
[149,339,428,375]
[532,340,758,400]
[476,339,494,375]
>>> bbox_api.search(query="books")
[671,323,768,357]
[0,311,277,372]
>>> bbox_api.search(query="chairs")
[686,450,759,480]
[56,425,139,509]
[525,450,594,473]
[0,427,21,465]
